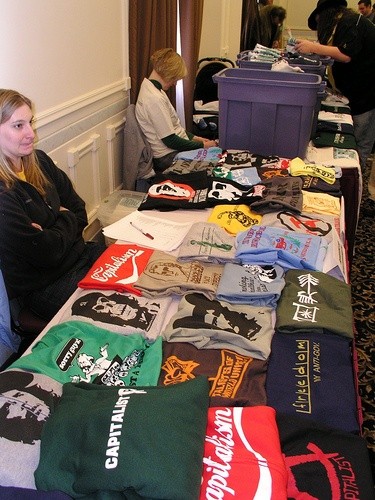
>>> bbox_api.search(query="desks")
[0,91,375,500]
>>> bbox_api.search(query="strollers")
[192,57,234,139]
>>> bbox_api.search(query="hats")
[308,0,347,30]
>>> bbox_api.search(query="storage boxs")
[212,50,334,160]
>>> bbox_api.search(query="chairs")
[123,104,155,192]
[0,271,46,368]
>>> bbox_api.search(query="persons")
[293,0,375,176]
[241,0,375,48]
[135,49,217,176]
[0,88,111,323]
